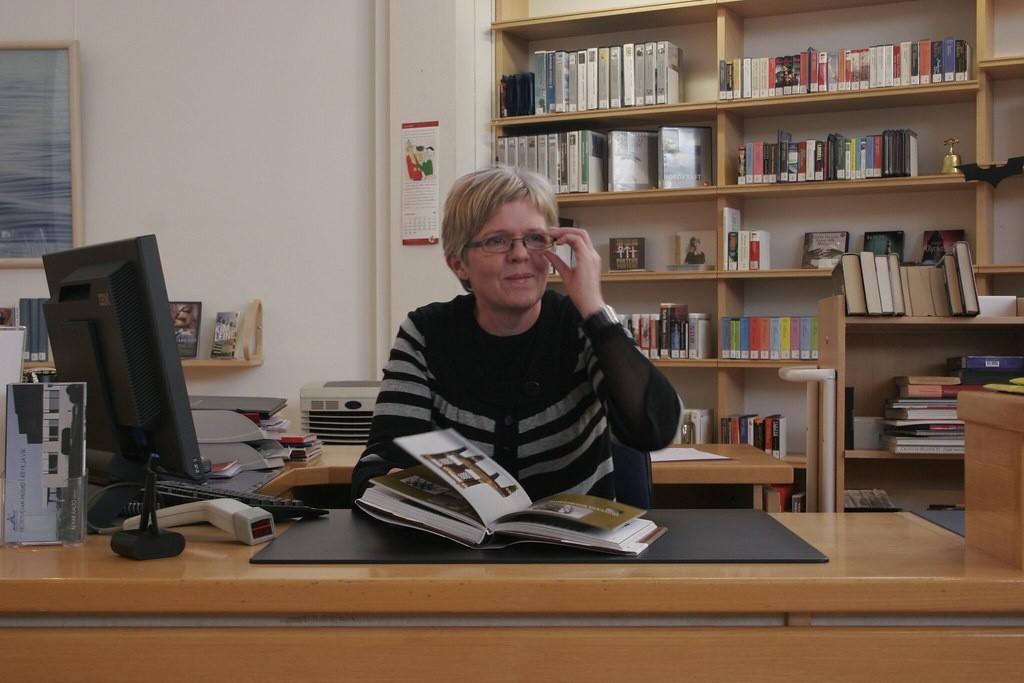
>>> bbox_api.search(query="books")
[763,484,806,513]
[169,300,203,360]
[496,125,712,193]
[844,354,1024,454]
[841,241,983,317]
[720,413,788,460]
[737,129,920,184]
[613,301,713,360]
[354,428,668,556]
[845,489,895,509]
[718,37,972,99]
[548,217,719,271]
[499,41,685,117]
[188,395,325,464]
[671,408,714,445]
[210,311,246,360]
[722,208,966,271]
[20,298,48,361]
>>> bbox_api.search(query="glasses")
[464,232,557,254]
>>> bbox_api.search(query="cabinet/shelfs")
[492,0,1024,513]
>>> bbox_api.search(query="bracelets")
[581,304,619,336]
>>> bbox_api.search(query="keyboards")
[141,478,331,522]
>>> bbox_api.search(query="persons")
[684,237,706,264]
[348,164,683,503]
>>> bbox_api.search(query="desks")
[1,512,1023,683]
[258,443,793,509]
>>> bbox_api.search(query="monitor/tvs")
[40,233,207,561]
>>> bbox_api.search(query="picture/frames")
[0,39,85,270]
